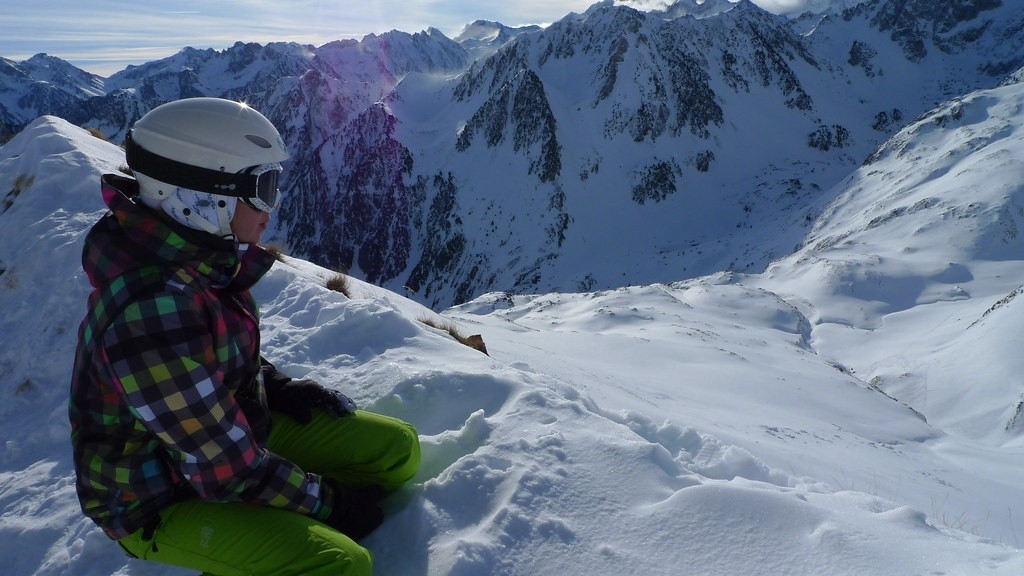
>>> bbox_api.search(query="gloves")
[271,379,357,425]
[325,482,388,539]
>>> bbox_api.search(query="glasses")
[240,162,283,214]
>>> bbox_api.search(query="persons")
[69,96,421,576]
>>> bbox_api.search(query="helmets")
[128,97,289,200]
